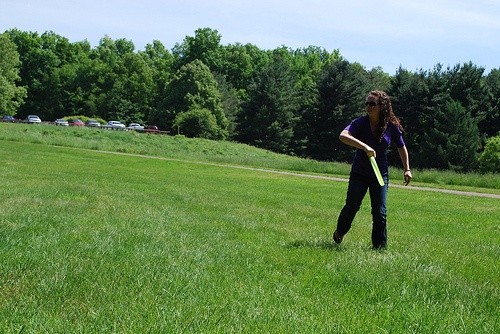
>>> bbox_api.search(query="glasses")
[365,101,380,106]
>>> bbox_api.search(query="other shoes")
[333,229,343,244]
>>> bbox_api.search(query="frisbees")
[371,156,385,186]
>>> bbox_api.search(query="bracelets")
[405,169,411,171]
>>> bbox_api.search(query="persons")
[333,89,412,253]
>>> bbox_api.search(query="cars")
[147,125,159,133]
[130,123,145,130]
[25,114,42,124]
[83,120,98,128]
[108,121,125,129]
[3,115,16,122]
[67,118,85,127]
[54,119,69,127]
